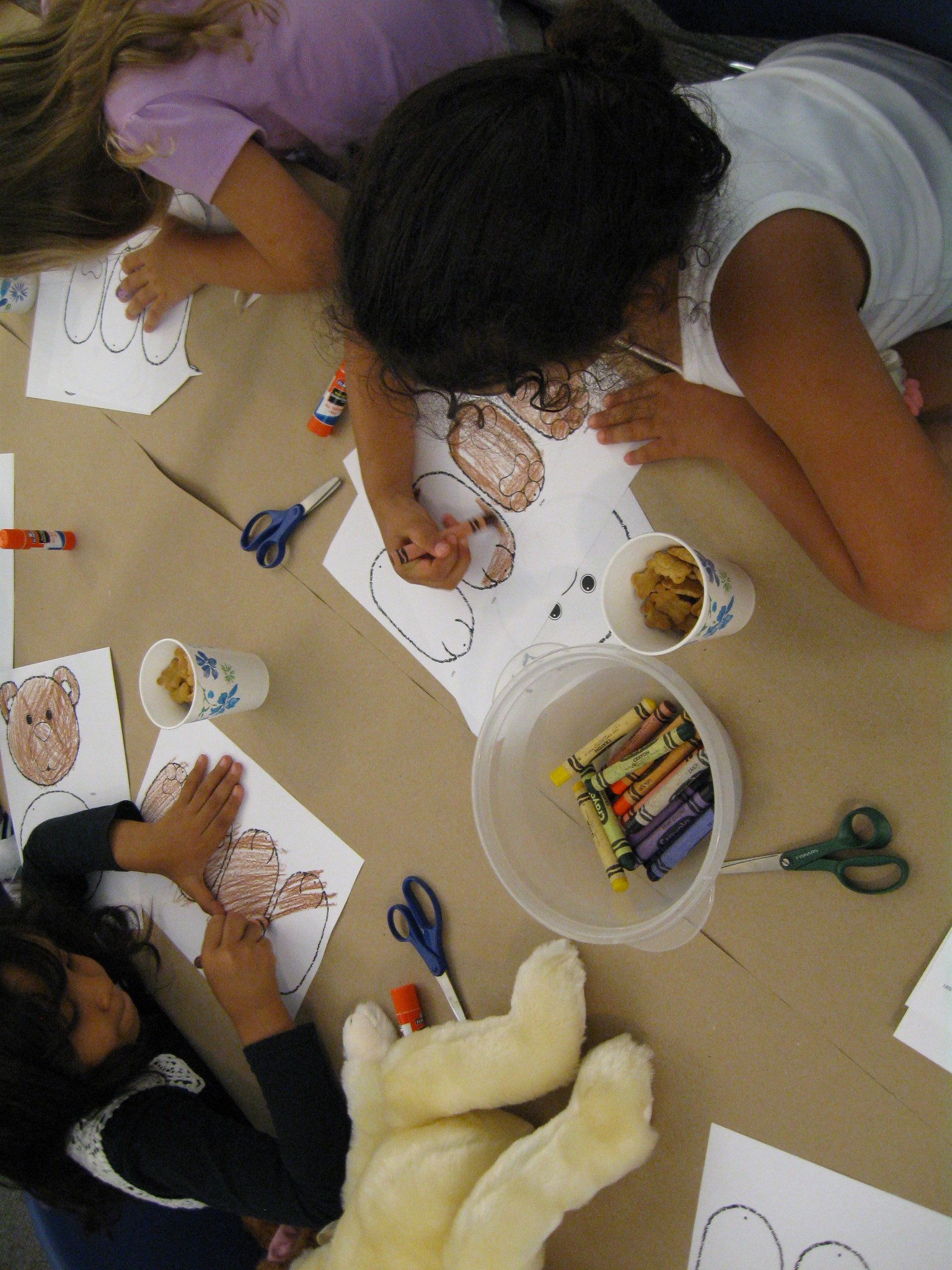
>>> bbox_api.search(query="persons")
[0,755,352,1269]
[329,1,952,634]
[0,0,546,332]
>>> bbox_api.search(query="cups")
[138,638,268,729]
[601,532,754,656]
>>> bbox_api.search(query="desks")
[0,0,952,1270]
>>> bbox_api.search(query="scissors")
[387,876,467,1023]
[720,807,911,896]
[241,477,341,569]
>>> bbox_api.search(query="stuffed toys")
[286,937,659,1270]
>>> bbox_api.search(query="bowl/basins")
[471,643,745,953]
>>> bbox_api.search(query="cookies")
[157,646,194,706]
[631,544,704,637]
